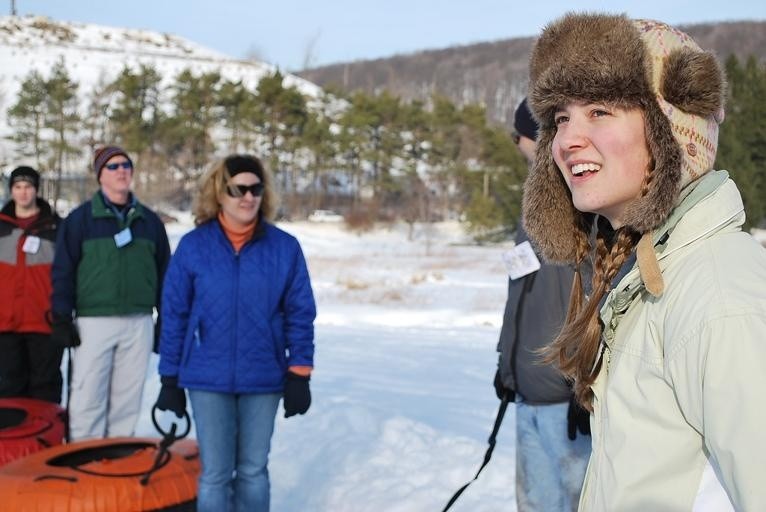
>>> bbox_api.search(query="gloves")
[48,313,80,349]
[568,394,590,440]
[284,370,311,418]
[493,370,514,403]
[156,375,186,419]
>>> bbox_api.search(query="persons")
[154,155,317,512]
[0,164,66,405]
[48,144,171,444]
[494,89,637,512]
[528,9,766,512]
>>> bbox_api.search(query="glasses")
[104,161,131,170]
[226,182,265,197]
[511,130,523,144]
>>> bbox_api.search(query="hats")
[514,95,539,142]
[10,167,38,192]
[520,10,725,264]
[95,147,133,185]
